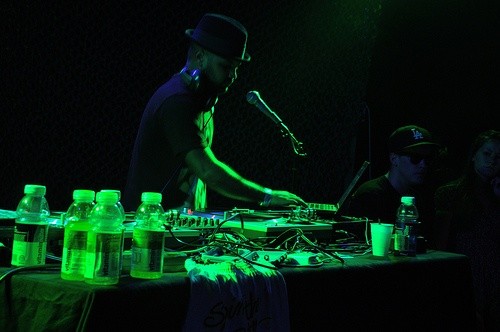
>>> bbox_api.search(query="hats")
[388,125,441,153]
[185,14,250,62]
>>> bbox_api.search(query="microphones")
[246,90,289,132]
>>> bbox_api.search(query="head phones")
[180,67,219,112]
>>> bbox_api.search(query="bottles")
[60,189,96,282]
[129,192,167,280]
[10,184,52,269]
[101,189,126,275]
[395,197,418,235]
[83,192,123,284]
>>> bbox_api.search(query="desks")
[0,232,466,332]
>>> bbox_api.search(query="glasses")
[395,151,433,165]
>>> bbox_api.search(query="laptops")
[277,160,370,217]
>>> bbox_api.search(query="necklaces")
[387,170,392,182]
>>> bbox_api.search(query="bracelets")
[261,187,272,207]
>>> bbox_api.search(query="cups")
[370,223,394,257]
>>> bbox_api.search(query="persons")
[126,12,308,251]
[344,123,500,321]
